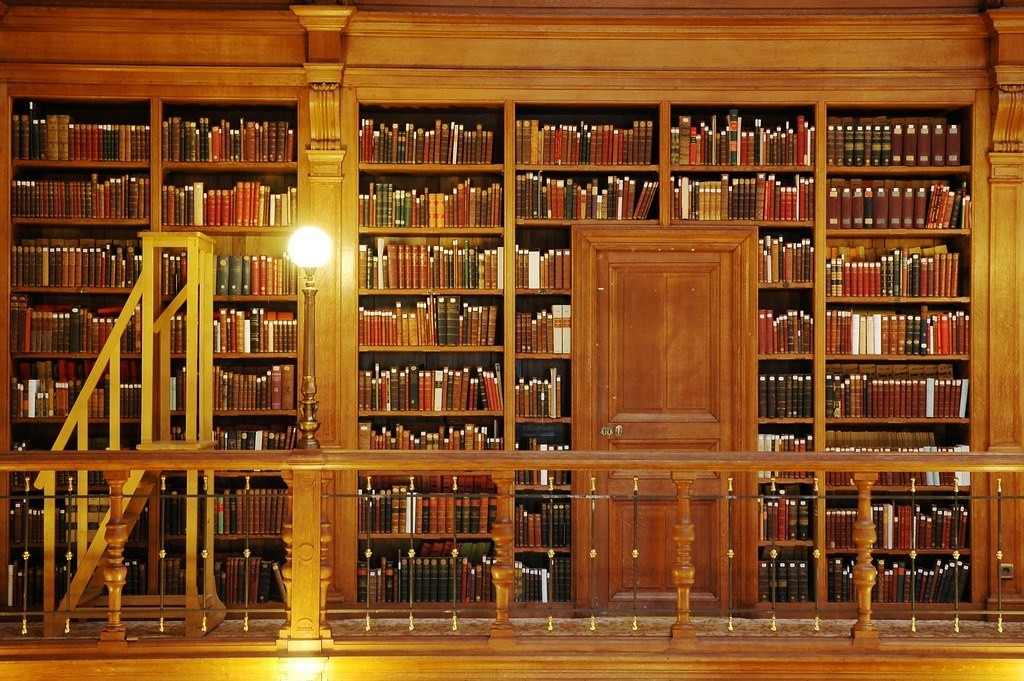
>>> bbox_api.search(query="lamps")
[286,225,331,448]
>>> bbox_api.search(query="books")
[358,111,971,604]
[7,101,297,606]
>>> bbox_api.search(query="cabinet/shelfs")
[2,62,988,619]
